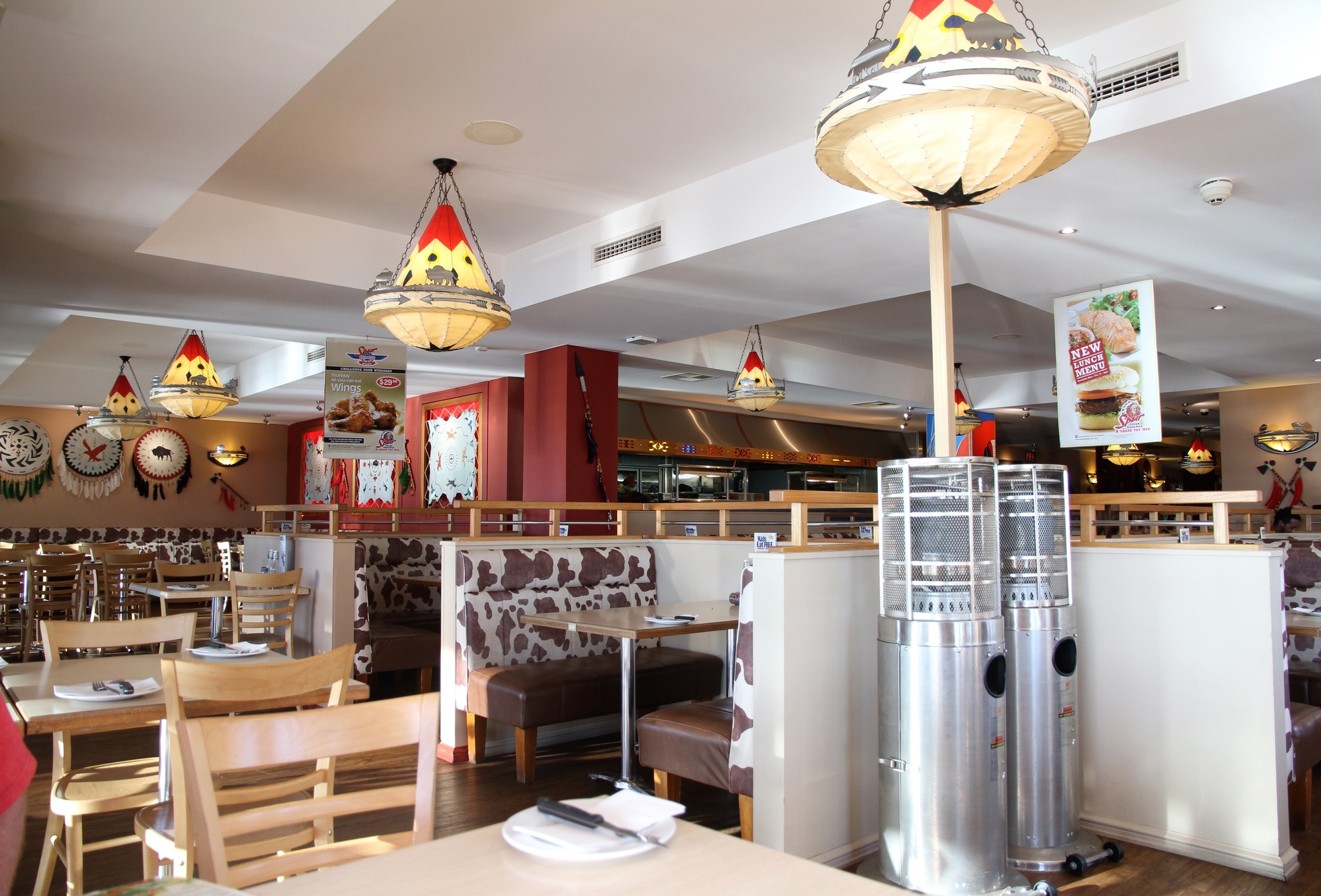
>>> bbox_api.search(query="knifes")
[537,797,668,850]
[660,615,695,620]
[117,678,133,694]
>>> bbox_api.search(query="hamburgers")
[1075,366,1141,429]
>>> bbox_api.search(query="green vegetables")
[1089,290,1140,329]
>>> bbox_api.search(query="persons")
[620,484,652,504]
[1270,506,1302,534]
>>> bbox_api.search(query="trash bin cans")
[853,454,1058,896]
[977,462,1125,877]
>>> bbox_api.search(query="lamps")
[1179,427,1219,474]
[726,324,786,412]
[362,158,512,353]
[900,418,910,429]
[813,0,1101,211]
[1022,407,1030,419]
[1086,473,1098,484]
[164,411,171,422]
[1149,479,1165,488]
[1253,421,1319,454]
[1182,403,1190,414]
[904,406,914,418]
[1102,443,1146,467]
[74,404,83,416]
[316,400,325,412]
[84,356,158,442]
[206,444,250,467]
[149,328,239,420]
[263,414,271,425]
[953,362,981,436]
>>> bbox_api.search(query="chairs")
[0,525,1321,896]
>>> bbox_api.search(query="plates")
[53,680,161,700]
[167,584,209,590]
[192,644,270,657]
[644,615,698,624]
[1292,607,1321,616]
[503,801,676,860]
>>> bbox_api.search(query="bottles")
[261,549,281,590]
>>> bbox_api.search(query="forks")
[653,613,686,621]
[204,637,250,654]
[179,583,197,588]
[92,679,122,695]
